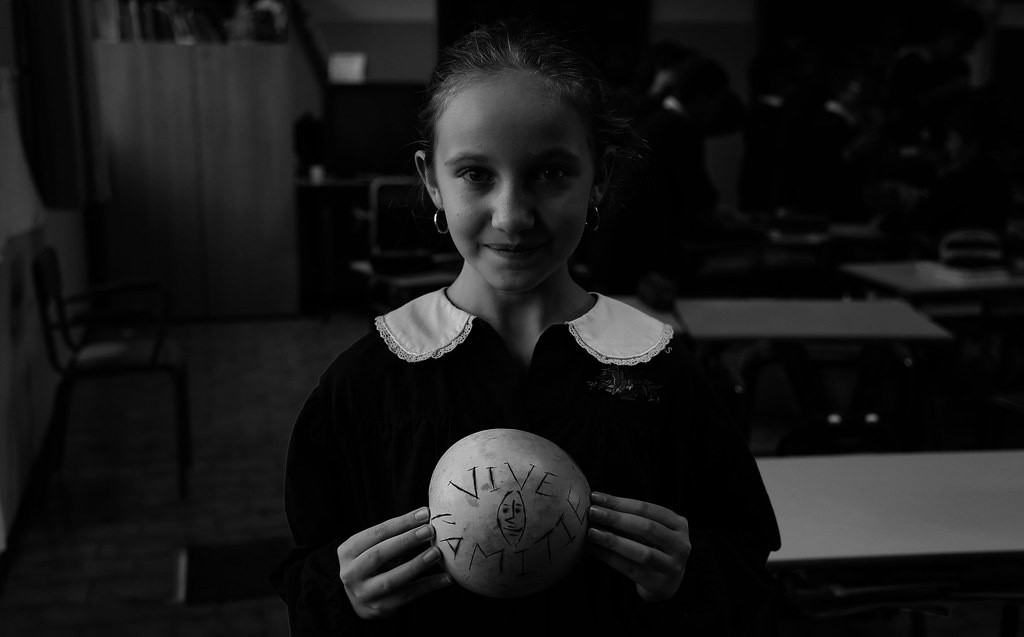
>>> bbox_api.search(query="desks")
[745,450,1024,572]
[840,261,1024,316]
[676,299,950,448]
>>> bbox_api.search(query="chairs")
[27,221,193,502]
[349,173,460,329]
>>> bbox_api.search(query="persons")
[283,41,780,637]
